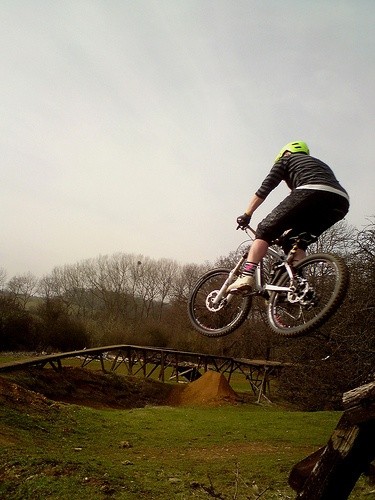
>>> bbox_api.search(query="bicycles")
[185,215,351,337]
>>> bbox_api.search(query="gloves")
[236,213,253,227]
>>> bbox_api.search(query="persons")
[225,141,350,295]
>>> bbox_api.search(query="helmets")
[273,141,310,162]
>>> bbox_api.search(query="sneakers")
[225,274,254,293]
